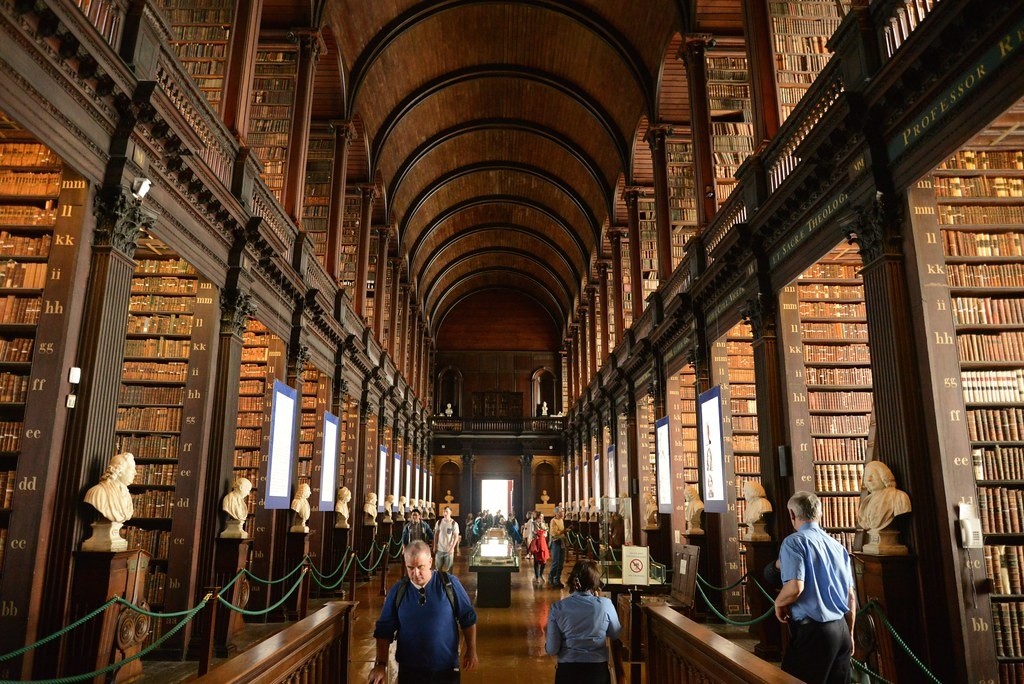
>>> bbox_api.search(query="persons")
[740,483,773,524]
[558,492,658,522]
[334,486,436,521]
[466,511,537,558]
[369,541,480,684]
[544,559,622,684]
[222,478,253,521]
[433,507,459,575]
[540,490,550,501]
[81,452,137,523]
[549,505,570,589]
[859,462,912,532]
[402,508,434,556]
[530,514,549,585]
[682,485,705,522]
[291,483,312,522]
[773,491,857,683]
[764,558,784,596]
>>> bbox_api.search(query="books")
[0,1,405,652]
[596,0,1023,682]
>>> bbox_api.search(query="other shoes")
[549,578,552,584]
[541,574,546,582]
[536,576,539,582]
[555,581,564,588]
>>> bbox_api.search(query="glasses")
[418,588,426,606]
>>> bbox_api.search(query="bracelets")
[375,661,386,666]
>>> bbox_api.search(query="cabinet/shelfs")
[0,1,436,664]
[562,0,1024,684]
